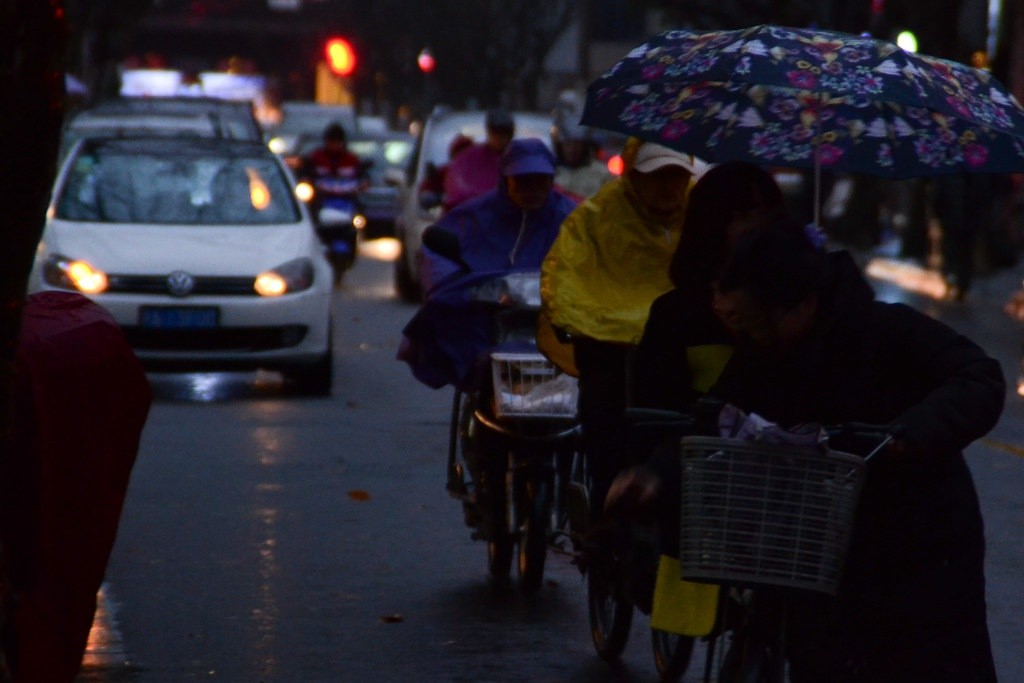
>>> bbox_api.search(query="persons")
[294,120,373,248]
[412,108,1006,682]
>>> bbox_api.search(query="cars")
[393,112,557,304]
[270,101,415,232]
[28,70,336,396]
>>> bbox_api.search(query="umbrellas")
[578,24,1023,231]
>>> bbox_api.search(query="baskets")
[489,349,580,419]
[679,431,864,597]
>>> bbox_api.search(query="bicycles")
[672,418,918,682]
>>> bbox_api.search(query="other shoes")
[477,489,506,539]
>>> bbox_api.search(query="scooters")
[420,225,580,623]
[295,157,376,284]
[550,321,696,683]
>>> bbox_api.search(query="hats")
[632,140,697,178]
[501,137,556,176]
[484,109,514,132]
